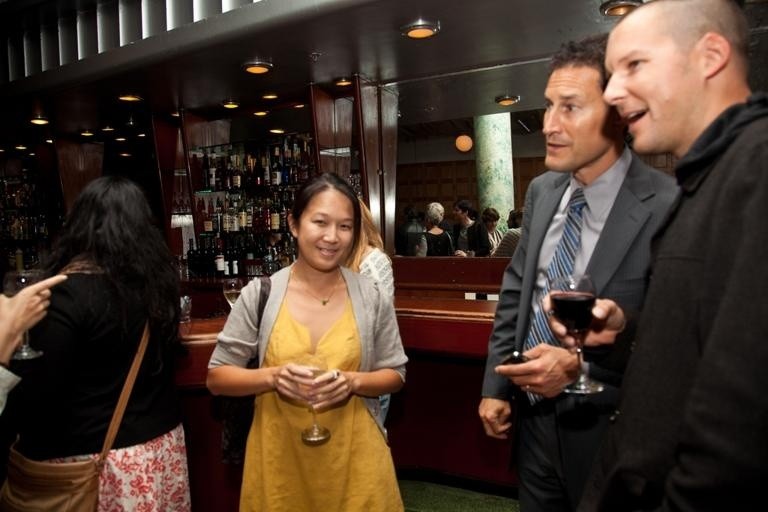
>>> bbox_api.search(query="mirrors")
[381,51,559,261]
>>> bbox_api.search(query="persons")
[473,36,682,511]
[342,196,397,437]
[22,176,196,512]
[206,170,412,512]
[540,0,768,512]
[0,274,67,420]
[392,194,526,307]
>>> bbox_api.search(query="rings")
[333,370,338,379]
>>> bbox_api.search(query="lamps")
[600,0,644,19]
[493,95,521,107]
[397,15,442,42]
[235,54,277,80]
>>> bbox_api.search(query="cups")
[178,296,191,324]
[224,278,243,309]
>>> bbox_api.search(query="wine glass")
[4,271,42,361]
[549,273,608,395]
[298,367,330,446]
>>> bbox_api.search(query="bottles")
[184,137,310,283]
[10,215,39,240]
[14,249,24,272]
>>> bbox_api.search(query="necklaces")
[291,270,342,306]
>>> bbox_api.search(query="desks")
[391,282,508,361]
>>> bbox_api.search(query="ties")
[522,189,588,407]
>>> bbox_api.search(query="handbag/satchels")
[0,447,101,512]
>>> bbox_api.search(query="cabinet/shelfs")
[189,132,312,279]
